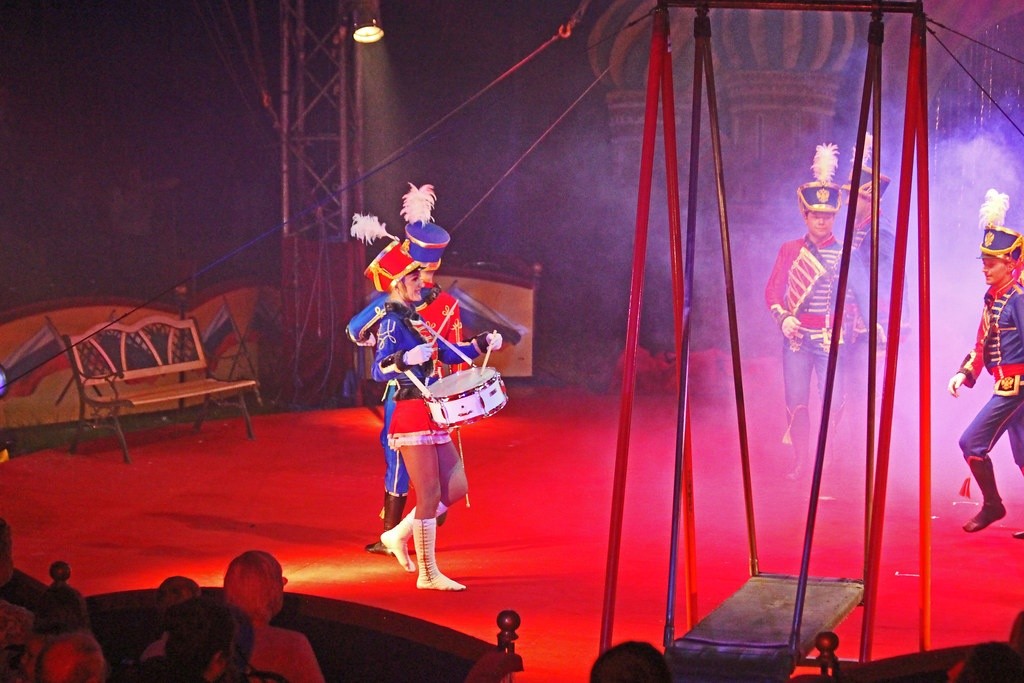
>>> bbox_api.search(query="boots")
[380,500,448,572]
[959,454,1006,532]
[1012,532,1024,539]
[781,404,810,482]
[413,517,466,590]
[818,402,846,501]
[365,486,408,557]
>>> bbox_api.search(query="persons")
[347,223,503,590]
[590,642,671,683]
[764,166,909,480]
[0,518,326,683]
[945,224,1024,538]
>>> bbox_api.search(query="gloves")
[486,333,503,351]
[356,332,376,346]
[403,342,434,365]
[877,321,886,346]
[948,373,967,398]
[781,316,802,338]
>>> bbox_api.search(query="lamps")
[352,0,385,45]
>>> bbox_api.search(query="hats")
[797,181,842,212]
[842,164,891,202]
[365,240,421,292]
[976,226,1024,262]
[405,221,450,271]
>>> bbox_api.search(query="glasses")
[282,576,288,589]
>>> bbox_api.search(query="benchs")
[60,316,261,465]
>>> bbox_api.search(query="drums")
[420,364,510,429]
